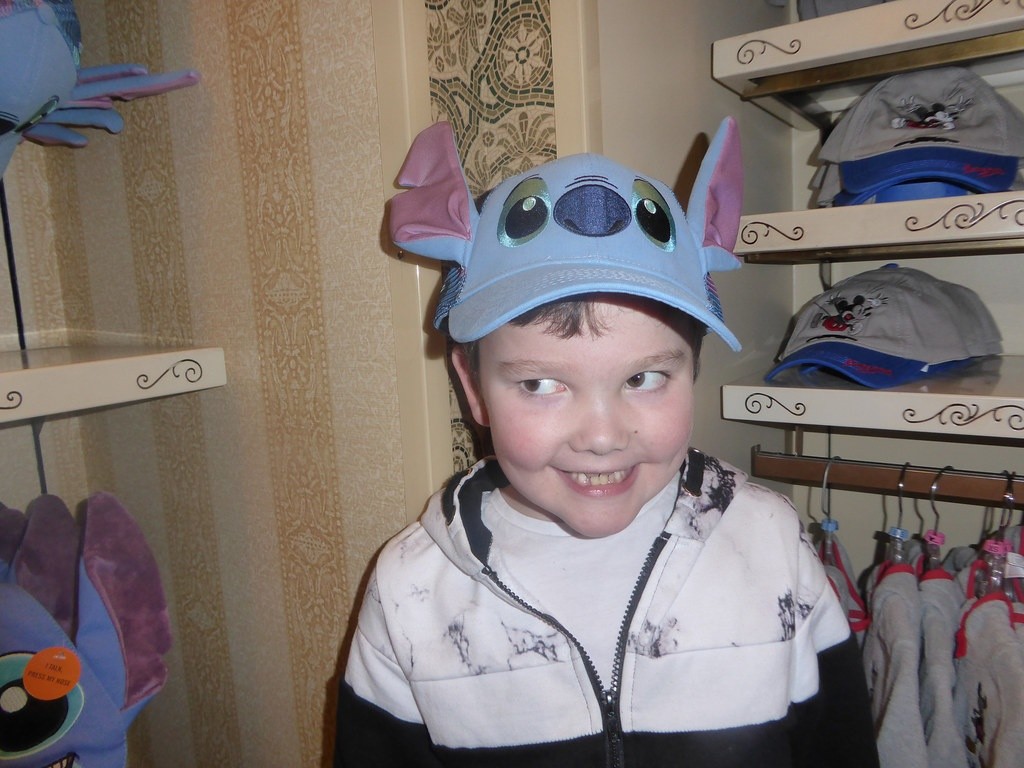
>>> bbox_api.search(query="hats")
[812,66,1024,207]
[764,262,1004,388]
[389,113,748,354]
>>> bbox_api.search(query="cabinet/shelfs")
[711,0,1024,450]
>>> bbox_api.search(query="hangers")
[811,455,1024,674]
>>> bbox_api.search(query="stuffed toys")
[0,490,174,767]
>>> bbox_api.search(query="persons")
[333,111,882,767]
